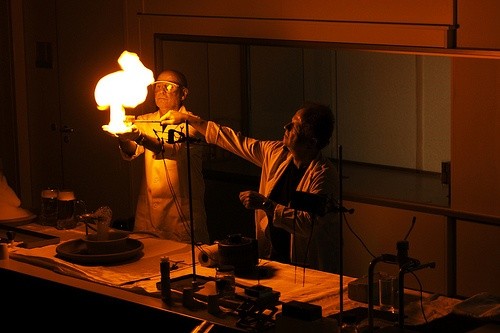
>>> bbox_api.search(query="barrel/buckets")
[217,233,258,274]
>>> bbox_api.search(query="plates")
[56,239,143,260]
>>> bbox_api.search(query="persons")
[102,69,209,244]
[161,103,338,268]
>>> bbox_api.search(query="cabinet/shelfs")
[159,38,451,173]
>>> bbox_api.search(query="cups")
[39,183,58,226]
[378,279,399,315]
[216,266,236,299]
[57,191,76,229]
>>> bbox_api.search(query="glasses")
[151,80,181,94]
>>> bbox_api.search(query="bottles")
[183,287,194,308]
[207,294,217,313]
[160,257,171,299]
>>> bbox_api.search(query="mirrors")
[153,35,452,207]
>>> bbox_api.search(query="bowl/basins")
[84,233,129,253]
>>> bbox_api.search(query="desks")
[0,214,500,333]
